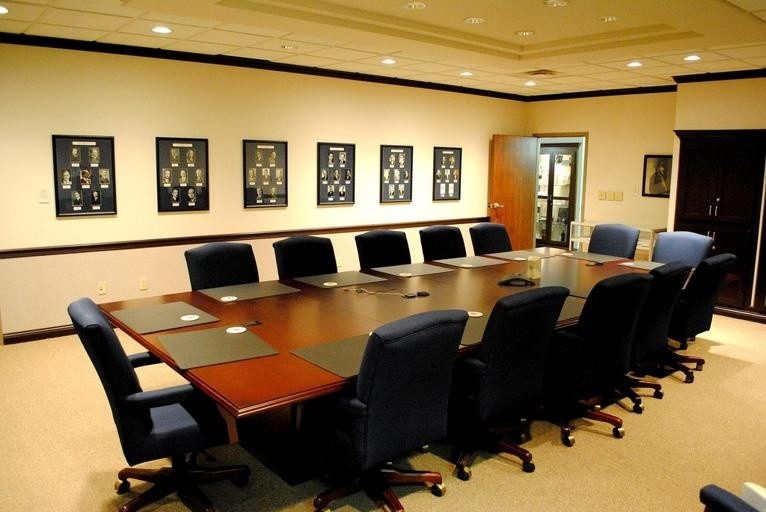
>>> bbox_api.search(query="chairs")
[313,308,470,512]
[420,225,467,262]
[469,222,513,257]
[654,231,714,269]
[618,261,693,414]
[354,229,414,270]
[184,242,259,293]
[438,285,570,482]
[535,273,655,447]
[659,254,737,384]
[587,223,640,259]
[69,298,250,512]
[272,233,338,279]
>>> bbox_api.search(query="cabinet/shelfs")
[567,223,667,262]
[673,130,765,317]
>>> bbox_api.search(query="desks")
[98,246,667,485]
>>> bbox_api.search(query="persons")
[162,149,202,201]
[648,160,669,196]
[61,147,108,203]
[247,151,282,199]
[435,156,458,183]
[321,153,351,196]
[384,155,408,195]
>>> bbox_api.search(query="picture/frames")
[154,136,209,213]
[378,143,414,204]
[640,153,669,199]
[240,137,288,207]
[432,146,461,202]
[50,133,118,217]
[314,141,355,205]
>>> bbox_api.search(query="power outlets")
[140,277,148,291]
[97,279,107,295]
